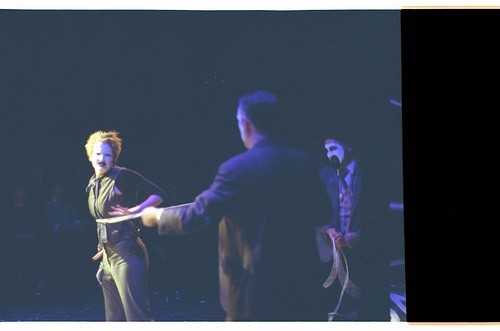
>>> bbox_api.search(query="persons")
[312,128,375,322]
[140,92,333,321]
[84,131,168,321]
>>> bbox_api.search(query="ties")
[337,171,350,192]
